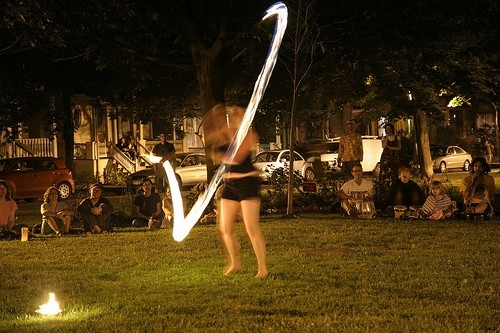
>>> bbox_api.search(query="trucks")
[319,135,384,179]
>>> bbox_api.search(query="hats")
[91,183,104,190]
[347,120,356,125]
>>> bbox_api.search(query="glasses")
[142,183,152,187]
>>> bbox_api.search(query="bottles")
[148,216,156,232]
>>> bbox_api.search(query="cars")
[250,148,315,184]
[417,146,473,173]
[124,152,207,191]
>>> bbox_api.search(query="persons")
[41,186,74,236]
[0,180,17,234]
[217,106,269,283]
[380,123,401,170]
[338,121,362,175]
[161,186,173,229]
[153,132,175,193]
[337,163,376,217]
[77,183,115,234]
[458,156,496,217]
[482,140,494,163]
[132,179,165,229]
[384,166,425,217]
[421,181,454,220]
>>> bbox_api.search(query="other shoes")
[100,229,108,234]
[57,231,72,238]
[347,207,359,219]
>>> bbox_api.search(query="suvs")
[0,157,75,202]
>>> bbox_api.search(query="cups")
[21,226,29,242]
